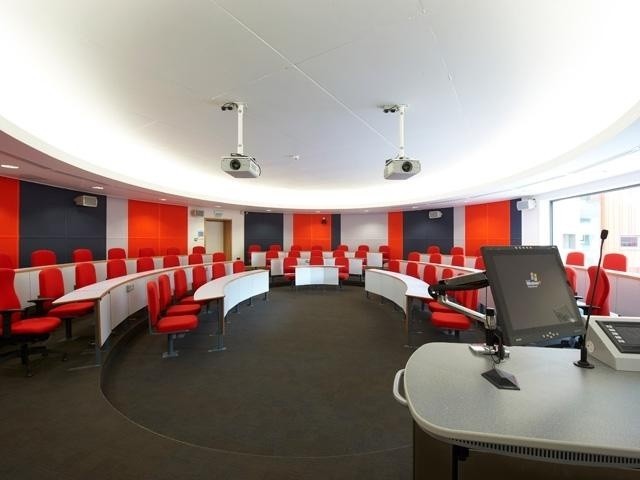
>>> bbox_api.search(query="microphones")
[574,229,609,368]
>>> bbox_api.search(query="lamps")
[214,208,224,217]
[516,198,536,211]
[428,210,443,219]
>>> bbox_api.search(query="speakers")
[429,211,441,219]
[517,199,535,210]
[214,210,223,216]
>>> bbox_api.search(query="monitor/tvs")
[583,315,640,372]
[480,245,586,346]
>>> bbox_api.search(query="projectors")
[220,156,261,178]
[384,158,421,180]
